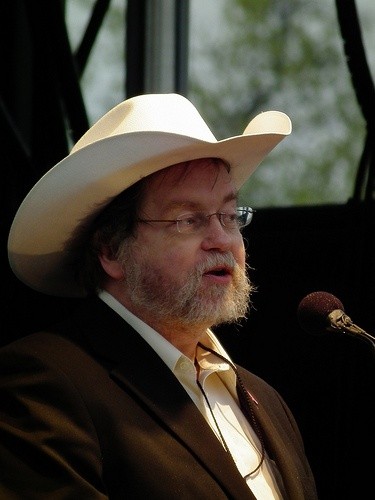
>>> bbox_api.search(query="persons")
[0,93,318,500]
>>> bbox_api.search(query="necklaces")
[194,341,265,481]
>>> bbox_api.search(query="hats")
[6,94,292,297]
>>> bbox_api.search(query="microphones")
[298,291,366,337]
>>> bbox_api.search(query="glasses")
[134,206,257,235]
